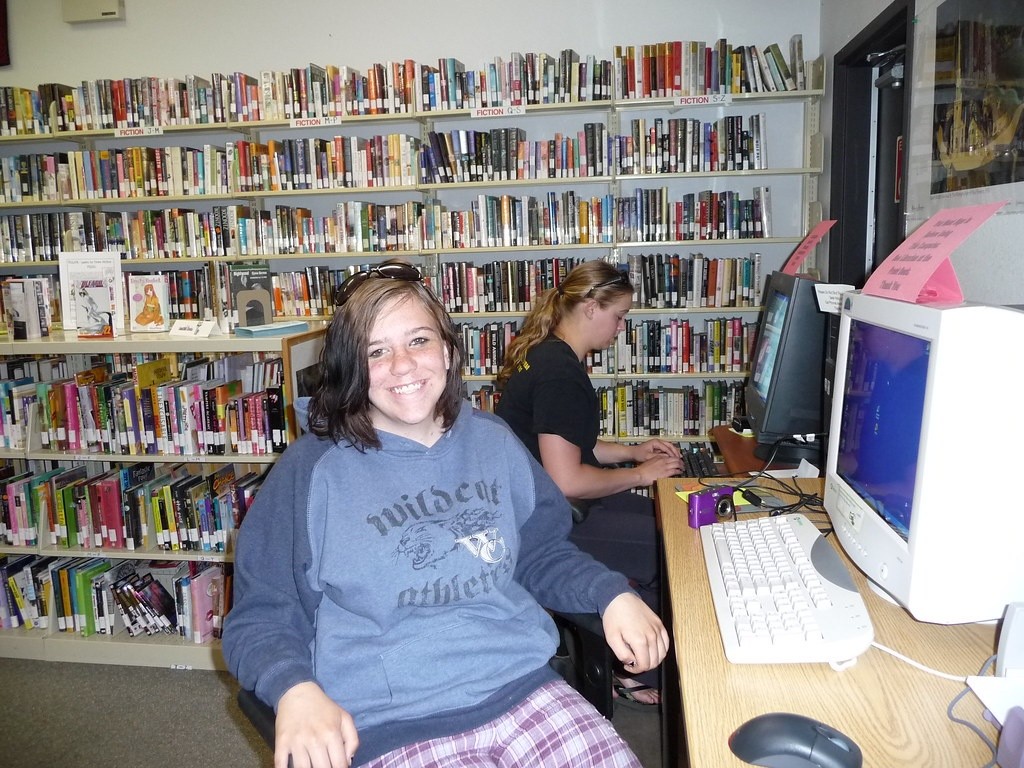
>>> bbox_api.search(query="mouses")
[729,712,864,768]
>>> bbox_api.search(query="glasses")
[588,271,630,297]
[332,263,437,315]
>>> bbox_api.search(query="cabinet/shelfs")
[0,31,829,673]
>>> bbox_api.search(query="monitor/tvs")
[744,270,823,468]
[822,287,1024,622]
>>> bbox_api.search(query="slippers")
[614,685,659,705]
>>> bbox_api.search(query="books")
[0,35,805,643]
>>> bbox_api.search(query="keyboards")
[698,513,875,663]
[673,440,722,478]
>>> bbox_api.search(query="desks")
[656,478,1000,768]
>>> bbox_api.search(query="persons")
[490,261,687,714]
[221,261,669,768]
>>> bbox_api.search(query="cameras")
[688,485,734,529]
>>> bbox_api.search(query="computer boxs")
[824,314,841,477]
